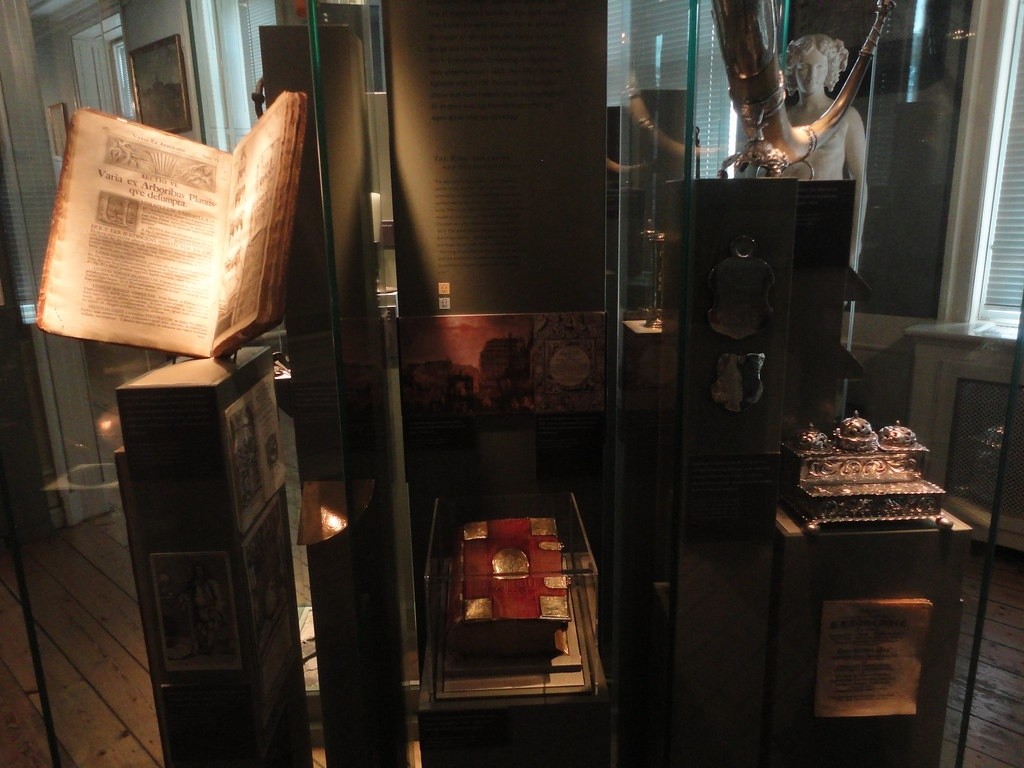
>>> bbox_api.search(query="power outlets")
[439,283,450,295]
[439,298,450,310]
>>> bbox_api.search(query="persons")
[762,34,868,269]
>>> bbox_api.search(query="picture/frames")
[126,34,193,135]
[46,103,68,157]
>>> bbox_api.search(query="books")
[445,517,572,658]
[814,599,933,718]
[224,370,287,535]
[37,90,309,358]
